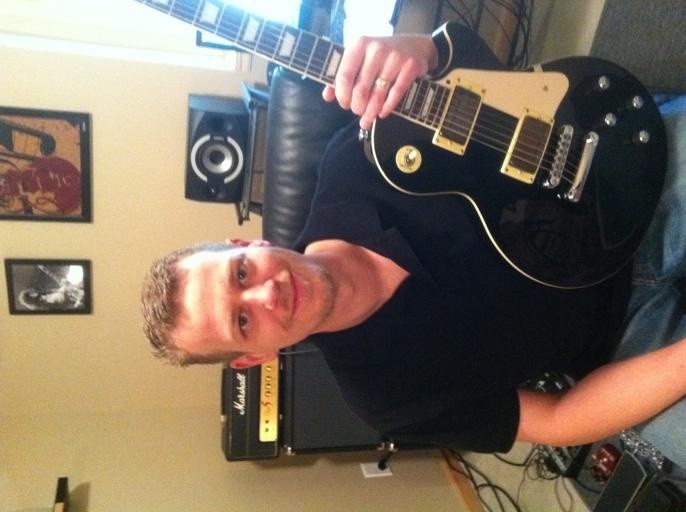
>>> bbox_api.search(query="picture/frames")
[0,104,93,224]
[4,259,91,314]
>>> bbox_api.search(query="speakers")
[185,92,248,202]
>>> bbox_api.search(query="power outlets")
[359,461,393,477]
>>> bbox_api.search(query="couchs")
[263,67,354,255]
